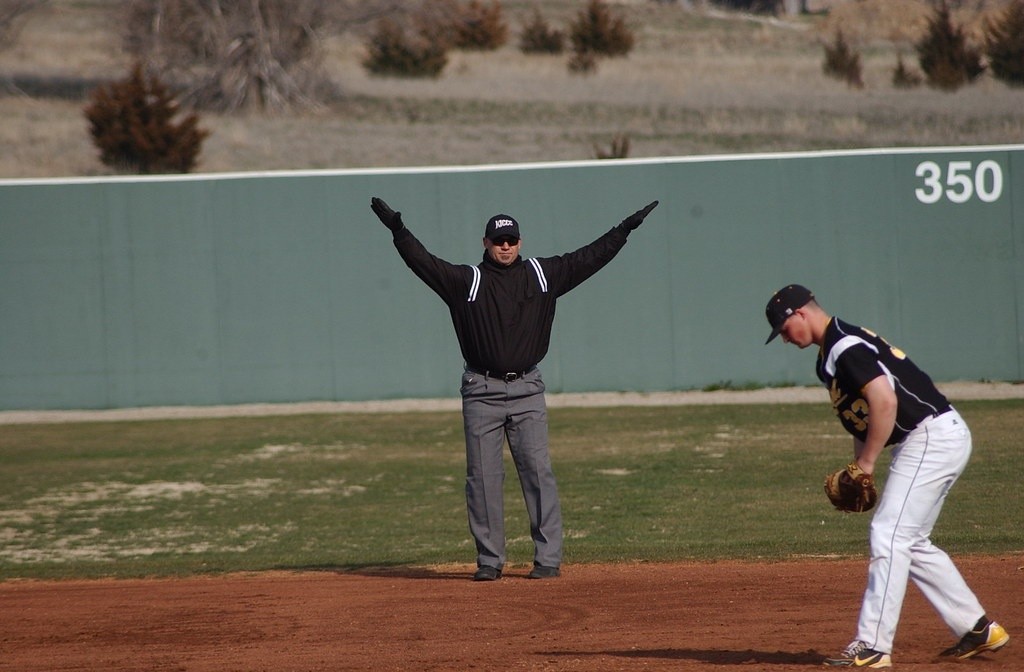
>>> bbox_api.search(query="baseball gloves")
[823,462,878,512]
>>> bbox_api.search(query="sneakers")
[474,565,503,580]
[530,563,560,579]
[931,621,1009,663]
[822,641,892,669]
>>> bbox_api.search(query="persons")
[764,284,1010,669]
[369,197,659,582]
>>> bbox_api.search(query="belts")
[467,363,536,381]
[931,405,952,418]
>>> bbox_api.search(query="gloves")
[371,197,405,234]
[621,201,658,231]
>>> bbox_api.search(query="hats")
[485,214,519,239]
[765,284,815,344]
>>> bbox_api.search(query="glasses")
[487,237,518,246]
